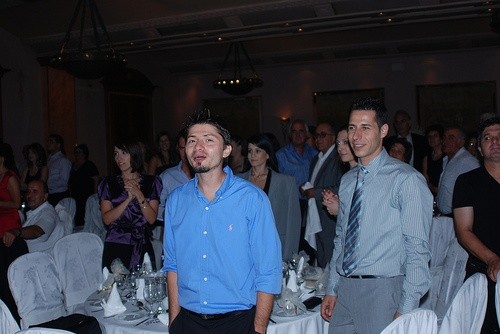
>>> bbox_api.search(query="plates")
[273,308,304,317]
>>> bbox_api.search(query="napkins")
[157,313,169,326]
[104,282,127,318]
[297,257,307,273]
[144,253,152,272]
[287,269,299,293]
[103,267,109,279]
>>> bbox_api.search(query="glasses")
[314,131,334,139]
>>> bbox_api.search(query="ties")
[342,167,369,277]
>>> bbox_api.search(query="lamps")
[63,0,116,79]
[211,42,262,96]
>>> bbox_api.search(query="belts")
[340,274,381,279]
[182,307,231,321]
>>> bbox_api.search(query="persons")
[163,118,283,334]
[320,97,433,334]
[0,108,495,329]
[451,117,500,334]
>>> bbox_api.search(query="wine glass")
[114,263,169,314]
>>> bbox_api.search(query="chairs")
[380,308,438,333]
[0,197,104,334]
[422,217,488,334]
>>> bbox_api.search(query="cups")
[284,304,298,315]
[305,266,322,289]
[282,258,297,275]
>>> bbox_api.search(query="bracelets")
[141,198,147,204]
[140,204,148,210]
[17,228,22,236]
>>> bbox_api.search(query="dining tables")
[84,262,330,334]
